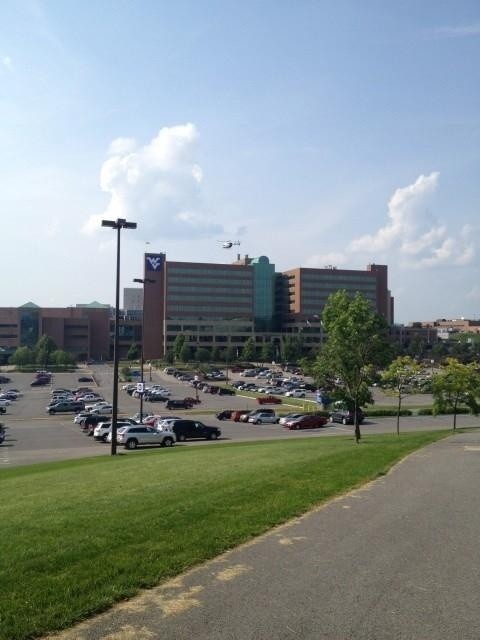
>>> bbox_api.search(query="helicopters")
[216,240,240,248]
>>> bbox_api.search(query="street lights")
[132,277,156,383]
[100,217,138,455]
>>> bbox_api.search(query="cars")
[0,369,51,444]
